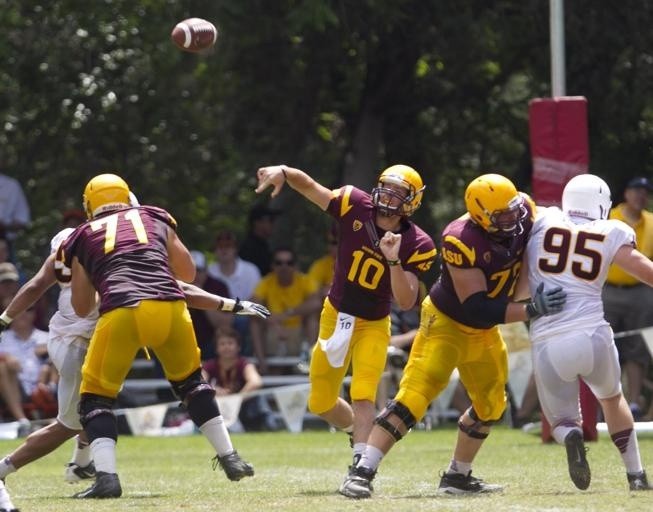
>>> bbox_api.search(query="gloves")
[526,282,567,318]
[231,296,272,320]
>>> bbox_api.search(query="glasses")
[274,259,295,266]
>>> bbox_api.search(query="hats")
[627,176,650,193]
[0,262,20,283]
[250,204,280,220]
[189,250,207,269]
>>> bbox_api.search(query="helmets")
[371,164,427,217]
[82,174,134,221]
[562,174,613,220]
[465,174,529,238]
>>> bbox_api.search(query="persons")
[337,171,568,499]
[253,164,437,492]
[0,186,272,511]
[2,171,652,432]
[525,171,653,493]
[55,172,256,500]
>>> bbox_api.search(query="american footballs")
[170,17,217,51]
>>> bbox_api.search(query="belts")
[604,280,643,289]
